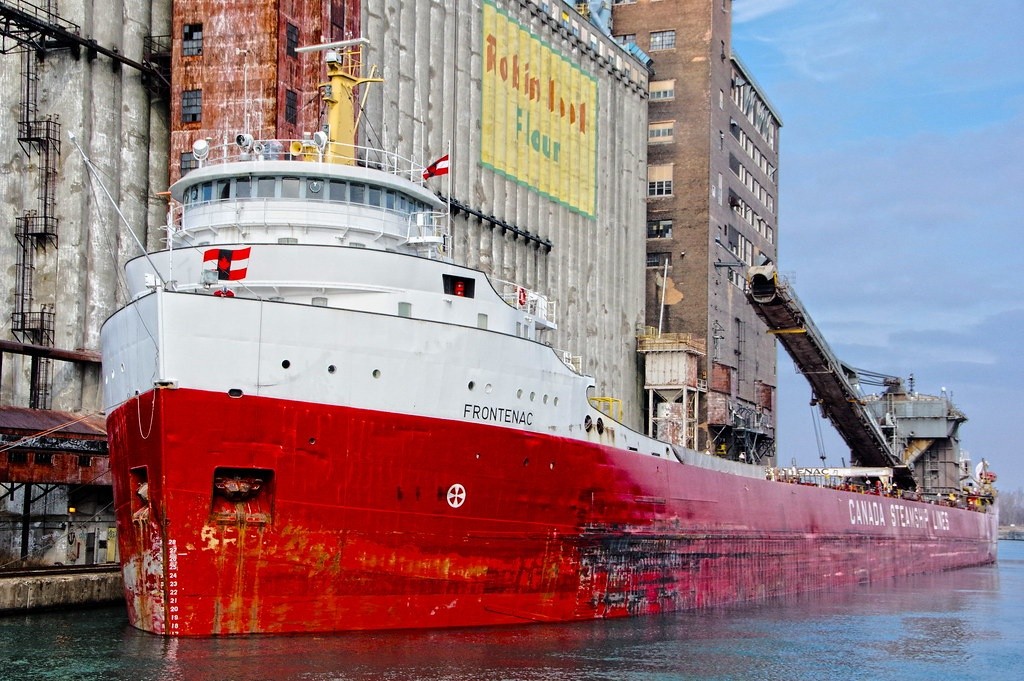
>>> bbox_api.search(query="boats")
[99,38,1000,640]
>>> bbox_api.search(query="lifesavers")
[518,288,527,305]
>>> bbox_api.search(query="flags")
[423,155,449,180]
